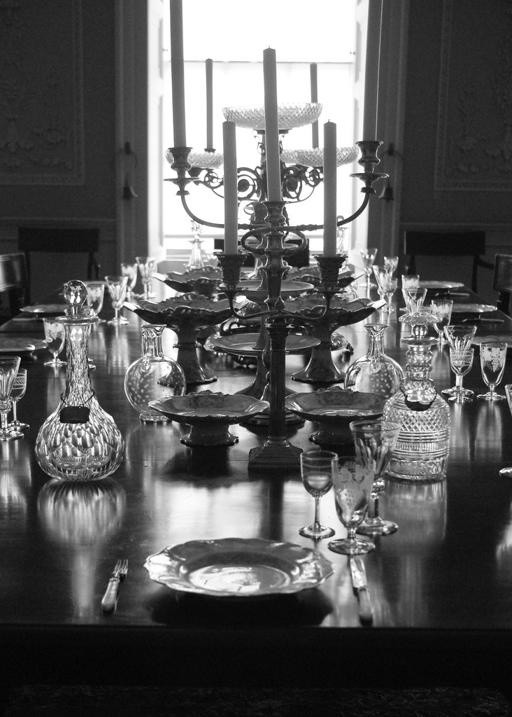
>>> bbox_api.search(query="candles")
[201,47,342,259]
[168,0,188,149]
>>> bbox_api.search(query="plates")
[437,302,498,315]
[20,303,69,315]
[144,535,334,597]
[418,278,464,289]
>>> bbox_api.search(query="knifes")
[348,555,374,623]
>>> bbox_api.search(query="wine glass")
[449,347,474,403]
[441,324,479,398]
[87,255,157,328]
[328,457,377,555]
[496,383,512,477]
[451,401,504,463]
[298,450,338,539]
[38,323,69,368]
[0,353,30,442]
[349,419,401,536]
[359,247,453,343]
[477,339,509,402]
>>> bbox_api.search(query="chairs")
[0,222,106,321]
[401,224,512,313]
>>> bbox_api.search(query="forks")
[100,557,129,614]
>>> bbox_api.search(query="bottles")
[125,324,187,424]
[378,484,448,626]
[37,475,128,625]
[344,323,406,395]
[380,324,452,483]
[33,279,124,484]
